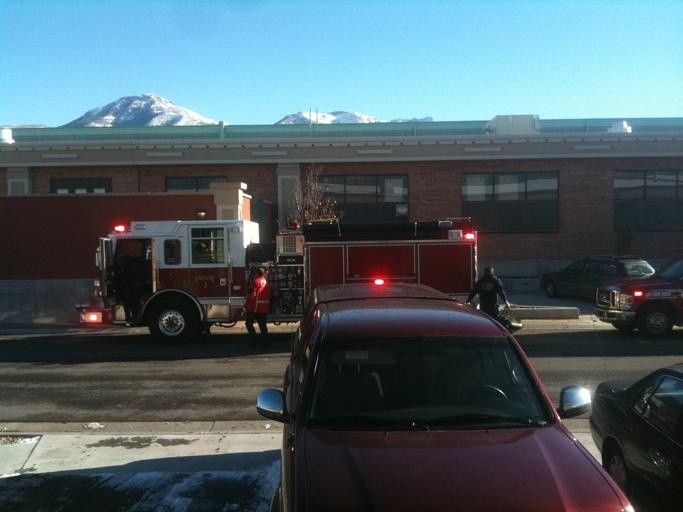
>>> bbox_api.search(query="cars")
[588,356,682,511]
[538,254,653,303]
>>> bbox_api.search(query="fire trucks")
[75,212,478,345]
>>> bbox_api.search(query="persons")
[246,267,273,337]
[469,267,512,317]
[116,253,148,321]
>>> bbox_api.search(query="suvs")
[591,254,682,340]
[251,279,637,512]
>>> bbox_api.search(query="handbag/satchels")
[496,306,524,335]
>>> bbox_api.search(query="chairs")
[320,374,384,405]
[425,359,490,404]
